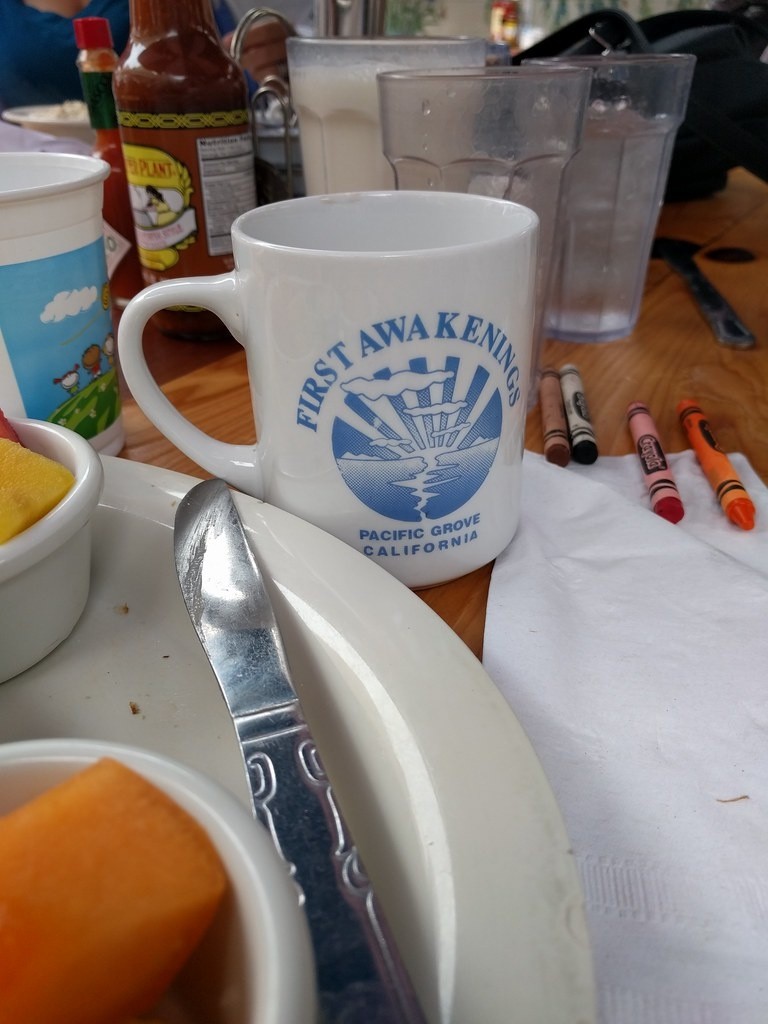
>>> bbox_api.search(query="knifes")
[660,240,757,350]
[173,478,425,1024]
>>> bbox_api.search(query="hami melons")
[0,757,230,1024]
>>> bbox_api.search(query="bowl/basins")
[0,414,103,686]
[2,104,96,146]
[1,737,319,1024]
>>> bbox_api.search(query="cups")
[0,153,127,461]
[283,34,697,343]
[114,189,542,590]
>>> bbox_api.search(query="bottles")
[110,0,260,341]
[71,17,145,310]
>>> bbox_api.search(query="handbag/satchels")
[508,6,753,199]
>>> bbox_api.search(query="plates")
[0,453,599,1022]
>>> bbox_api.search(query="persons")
[0,0,291,114]
